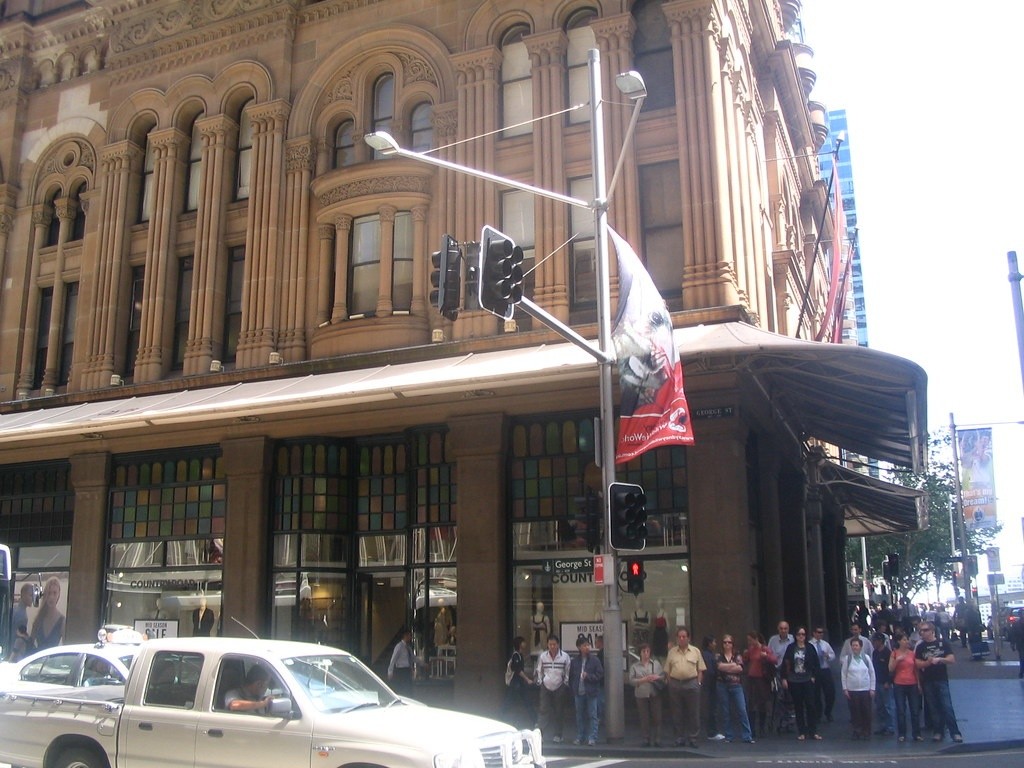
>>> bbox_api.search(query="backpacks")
[505,651,521,687]
[16,635,38,658]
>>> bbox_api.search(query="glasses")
[920,629,931,632]
[722,641,733,644]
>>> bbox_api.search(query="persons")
[498,634,605,745]
[433,598,457,656]
[320,614,329,641]
[1008,610,1024,679]
[150,598,170,620]
[627,617,963,746]
[847,597,970,647]
[631,598,651,647]
[83,660,120,687]
[530,602,550,683]
[8,625,31,663]
[148,660,191,706]
[192,598,214,637]
[10,576,66,649]
[388,632,414,699]
[223,664,275,715]
[652,597,671,661]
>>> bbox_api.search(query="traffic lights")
[882,553,899,582]
[608,482,648,552]
[626,558,648,595]
[430,234,461,321]
[575,492,600,553]
[479,225,525,322]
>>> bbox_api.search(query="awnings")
[752,358,920,478]
[812,456,929,540]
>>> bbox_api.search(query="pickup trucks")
[0,636,547,768]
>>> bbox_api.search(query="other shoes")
[797,735,805,741]
[690,742,698,749]
[587,740,599,746]
[573,739,586,745]
[952,735,963,742]
[553,736,564,743]
[874,729,894,737]
[931,734,944,741]
[653,742,665,748]
[670,742,685,747]
[707,734,725,740]
[809,734,822,740]
[913,736,925,741]
[898,736,906,741]
[639,742,650,748]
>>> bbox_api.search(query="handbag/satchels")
[654,679,671,690]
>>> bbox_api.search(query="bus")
[0,544,45,663]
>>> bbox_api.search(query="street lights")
[364,48,647,743]
[950,412,1024,603]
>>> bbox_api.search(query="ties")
[817,641,824,666]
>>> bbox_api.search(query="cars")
[986,604,1024,641]
[530,646,655,686]
[0,625,201,701]
